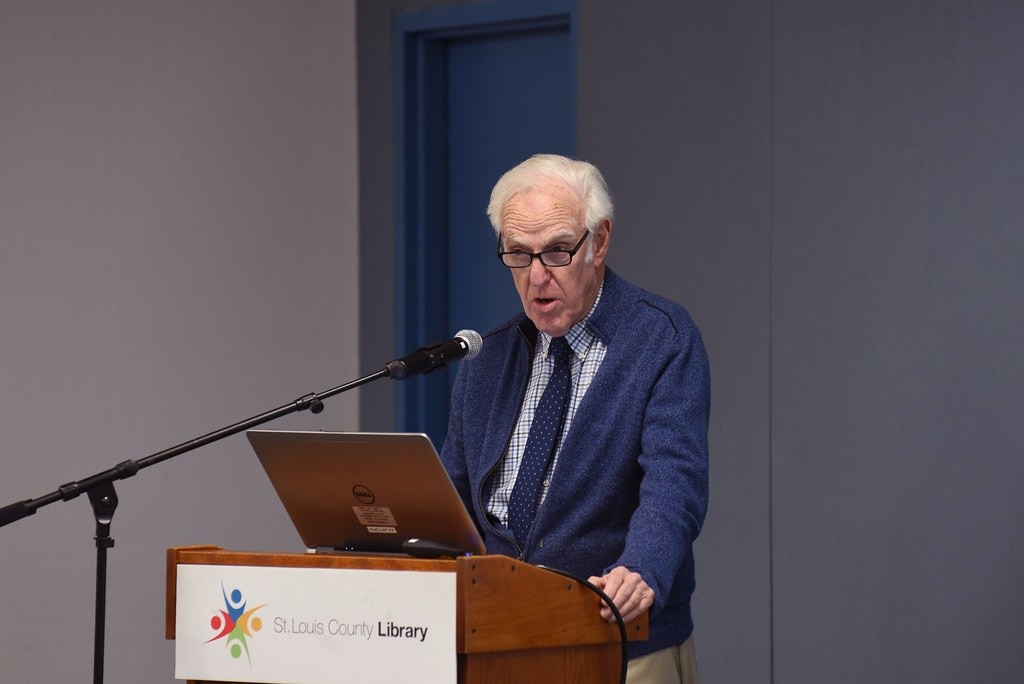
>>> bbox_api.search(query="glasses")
[497,230,589,269]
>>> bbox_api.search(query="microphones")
[391,330,483,381]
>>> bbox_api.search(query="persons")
[441,154,710,684]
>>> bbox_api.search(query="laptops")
[245,430,488,555]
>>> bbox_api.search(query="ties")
[508,336,574,553]
